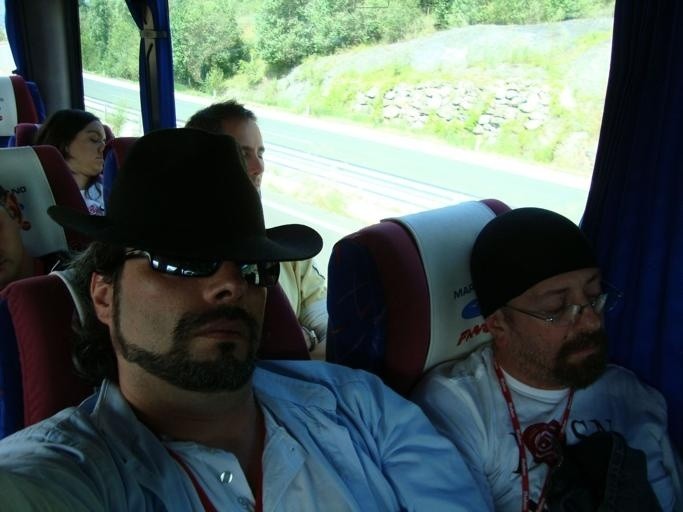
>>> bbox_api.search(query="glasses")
[501,283,626,332]
[120,245,282,290]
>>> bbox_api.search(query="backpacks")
[539,427,665,512]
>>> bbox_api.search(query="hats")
[45,126,324,265]
[469,205,605,319]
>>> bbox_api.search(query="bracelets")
[302,326,319,354]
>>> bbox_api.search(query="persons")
[412,206,681,511]
[182,99,339,359]
[0,122,488,510]
[0,183,78,440]
[39,111,109,216]
